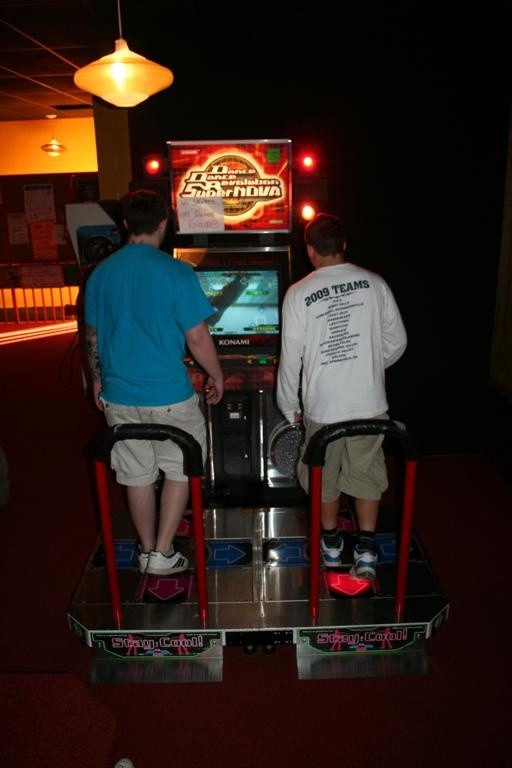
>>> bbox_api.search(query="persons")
[83,189,225,577]
[276,213,410,582]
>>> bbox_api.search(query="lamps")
[73,1,175,109]
[41,114,68,156]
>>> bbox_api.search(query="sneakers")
[136,543,189,577]
[352,544,378,581]
[320,536,345,568]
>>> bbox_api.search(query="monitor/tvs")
[193,269,279,338]
